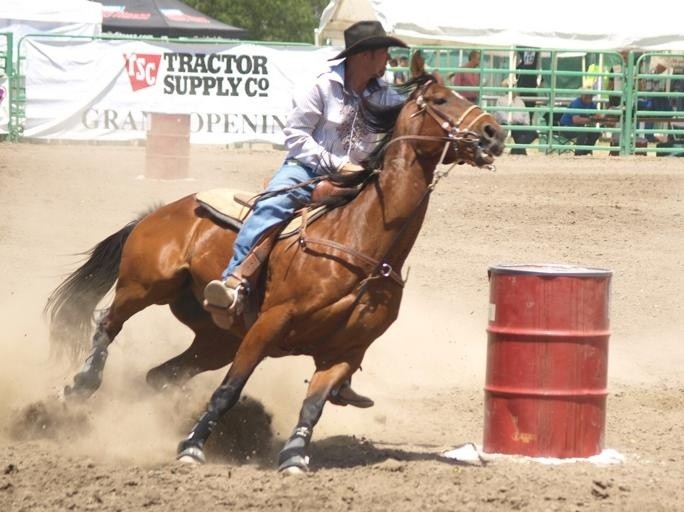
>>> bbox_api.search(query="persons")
[205,21,405,409]
[387,47,480,107]
[492,47,683,156]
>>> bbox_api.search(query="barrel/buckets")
[484,264,611,458]
[145,112,190,180]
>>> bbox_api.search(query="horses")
[41,46,511,478]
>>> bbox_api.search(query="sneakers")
[204,279,237,330]
[327,387,373,407]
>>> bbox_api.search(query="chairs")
[533,107,575,155]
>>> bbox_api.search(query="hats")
[501,77,519,85]
[328,21,408,61]
